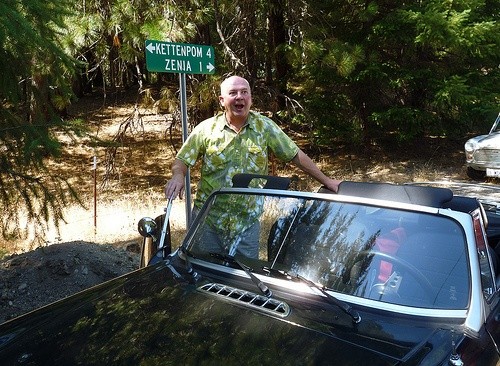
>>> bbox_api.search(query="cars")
[0,172,500,366]
[464,112,500,180]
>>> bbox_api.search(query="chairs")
[281,224,457,309]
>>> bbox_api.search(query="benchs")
[337,181,453,208]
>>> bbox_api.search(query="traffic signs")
[145,39,216,75]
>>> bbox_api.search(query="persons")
[164,75,345,259]
